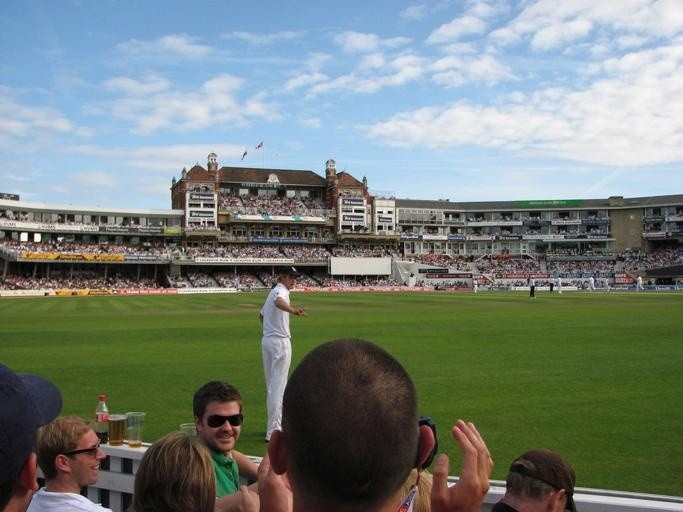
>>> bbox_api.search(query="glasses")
[64,439,101,458]
[201,413,243,429]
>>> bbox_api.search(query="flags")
[242,150,247,160]
[255,141,263,150]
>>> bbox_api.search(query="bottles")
[94,395,109,446]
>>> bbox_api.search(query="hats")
[280,265,302,279]
[508,448,579,512]
[0,363,63,486]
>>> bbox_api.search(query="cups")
[180,423,197,437]
[109,414,125,447]
[126,412,145,448]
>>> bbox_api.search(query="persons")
[259,267,308,442]
[0,363,62,512]
[27,417,112,512]
[127,432,260,512]
[193,381,259,512]
[492,449,577,512]
[0,194,683,294]
[403,468,434,512]
[269,337,493,512]
